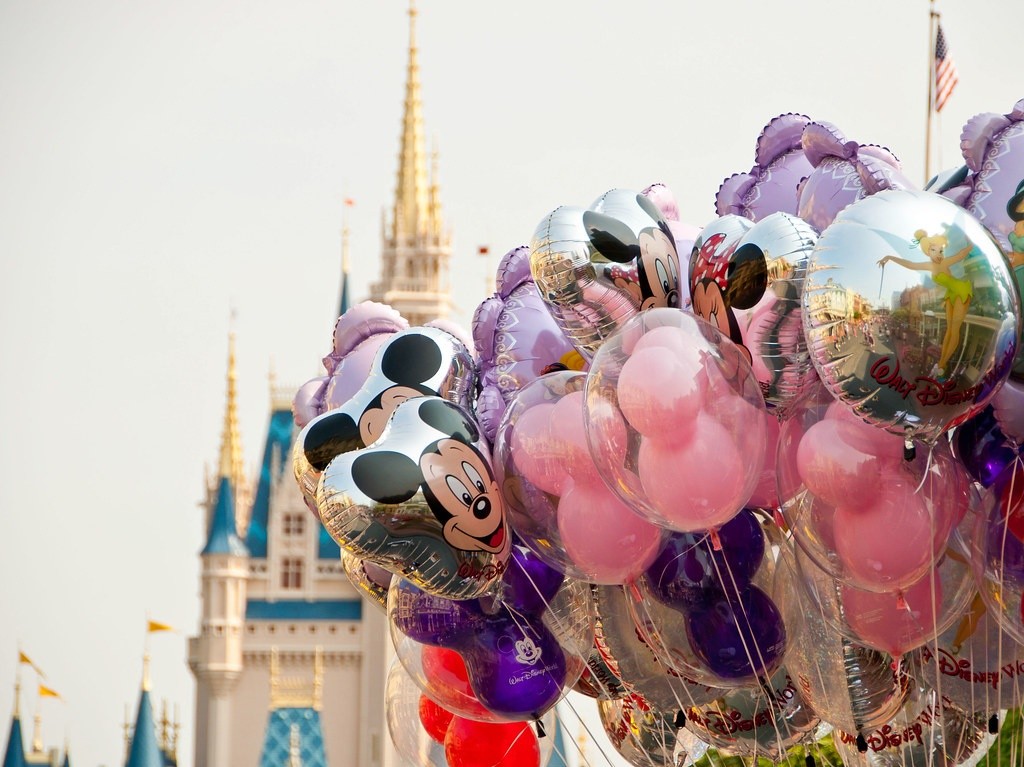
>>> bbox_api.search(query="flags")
[931,15,960,117]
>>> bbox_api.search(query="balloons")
[293,97,1024,767]
